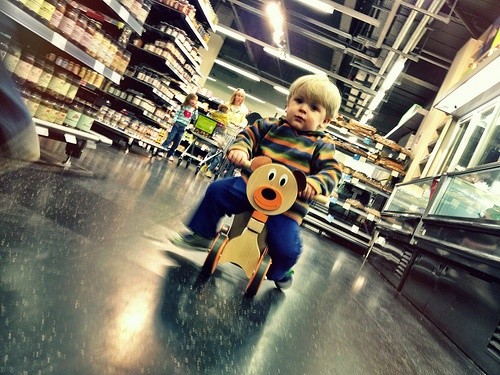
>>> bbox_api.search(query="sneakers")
[158,152,174,161]
[273,269,294,289]
[200,166,212,178]
[169,233,211,252]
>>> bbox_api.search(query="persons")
[151,93,198,160]
[199,88,251,178]
[167,74,343,290]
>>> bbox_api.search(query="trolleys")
[178,112,243,179]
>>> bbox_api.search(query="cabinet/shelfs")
[307,119,411,254]
[1,0,153,158]
[95,0,219,159]
